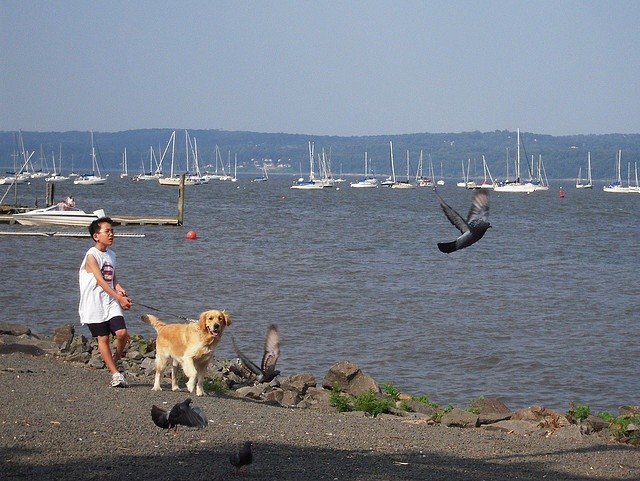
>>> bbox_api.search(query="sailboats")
[45,143,70,182]
[437,162,445,185]
[414,150,430,182]
[175,136,211,184]
[120,147,128,179]
[231,153,238,182]
[380,141,396,185]
[203,144,227,180]
[308,146,335,187]
[290,141,324,190]
[253,163,269,182]
[575,151,594,188]
[492,128,540,192]
[155,130,201,185]
[154,143,164,178]
[335,162,347,183]
[603,149,640,193]
[417,153,436,186]
[456,158,477,187]
[67,154,79,177]
[392,150,413,189]
[350,152,379,188]
[74,128,110,184]
[132,146,161,182]
[0,131,50,184]
[467,155,494,188]
[220,150,233,181]
[530,154,551,191]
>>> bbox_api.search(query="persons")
[79,217,134,389]
[69,198,76,207]
[56,200,68,211]
[66,195,73,206]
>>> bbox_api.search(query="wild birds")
[433,182,492,253]
[150,397,207,435]
[227,322,282,391]
[226,439,253,475]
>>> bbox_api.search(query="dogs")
[140,309,231,400]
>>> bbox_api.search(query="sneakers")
[113,356,127,378]
[111,371,127,388]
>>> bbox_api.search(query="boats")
[12,200,106,226]
